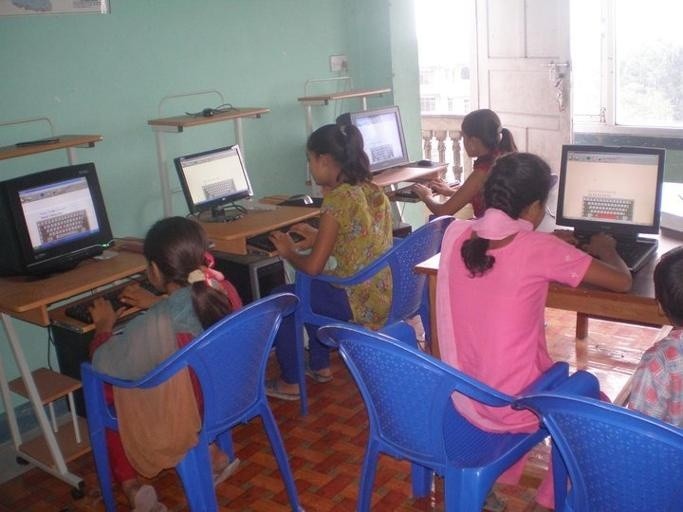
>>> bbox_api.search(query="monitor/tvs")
[334,105,411,179]
[555,142,665,242]
[174,143,254,222]
[0,161,117,281]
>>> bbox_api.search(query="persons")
[436,151,633,511]
[265,112,394,402]
[87,216,244,512]
[409,109,520,221]
[628,246,683,431]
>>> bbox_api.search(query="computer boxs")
[390,220,413,240]
[50,324,96,418]
[214,262,285,306]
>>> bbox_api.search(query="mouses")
[416,157,431,166]
[284,193,314,205]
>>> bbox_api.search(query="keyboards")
[566,238,656,274]
[63,279,161,322]
[247,214,322,252]
[394,178,459,200]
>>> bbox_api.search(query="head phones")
[184,100,243,117]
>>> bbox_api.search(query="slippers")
[133,484,167,512]
[264,377,300,401]
[303,359,334,384]
[213,458,241,488]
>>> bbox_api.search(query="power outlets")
[330,54,347,71]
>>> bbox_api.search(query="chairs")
[80,293,305,512]
[517,369,683,512]
[293,215,456,416]
[316,320,569,512]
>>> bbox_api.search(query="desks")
[415,204,683,365]
[1,118,215,499]
[147,90,321,302]
[297,76,447,236]
